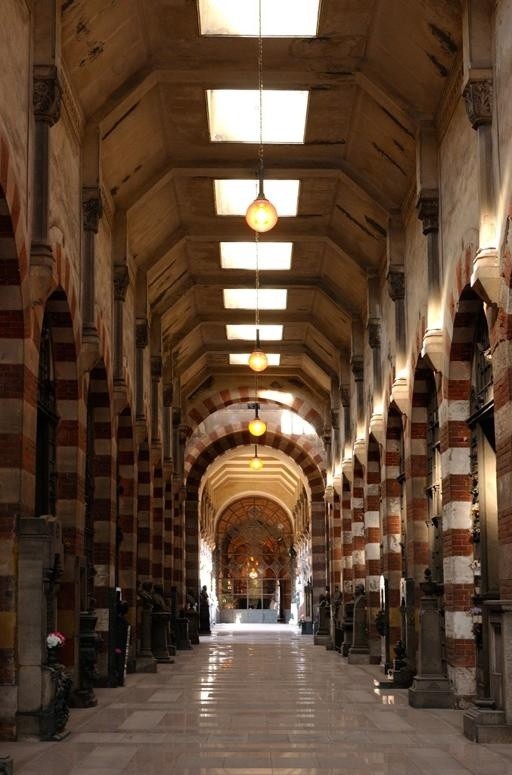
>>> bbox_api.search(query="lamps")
[246,556,259,579]
[244,0,279,234]
[248,161,269,372]
[250,444,262,470]
[248,372,267,437]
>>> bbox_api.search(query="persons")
[316,586,330,607]
[152,583,166,611]
[332,585,342,606]
[353,584,368,608]
[138,579,154,604]
[200,584,208,599]
